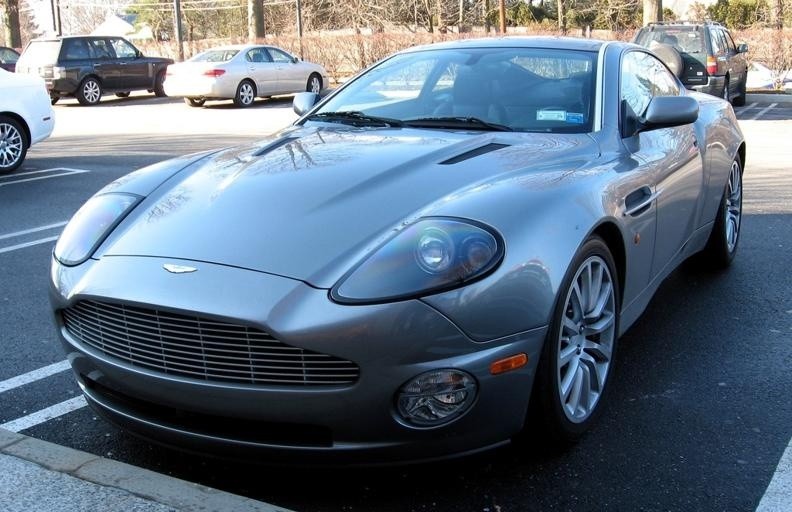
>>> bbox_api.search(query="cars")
[47,39,746,492]
[162,43,331,108]
[0,36,174,177]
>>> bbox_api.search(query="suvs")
[633,21,748,109]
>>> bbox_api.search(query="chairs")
[253,53,263,62]
[433,72,508,125]
[660,35,682,52]
